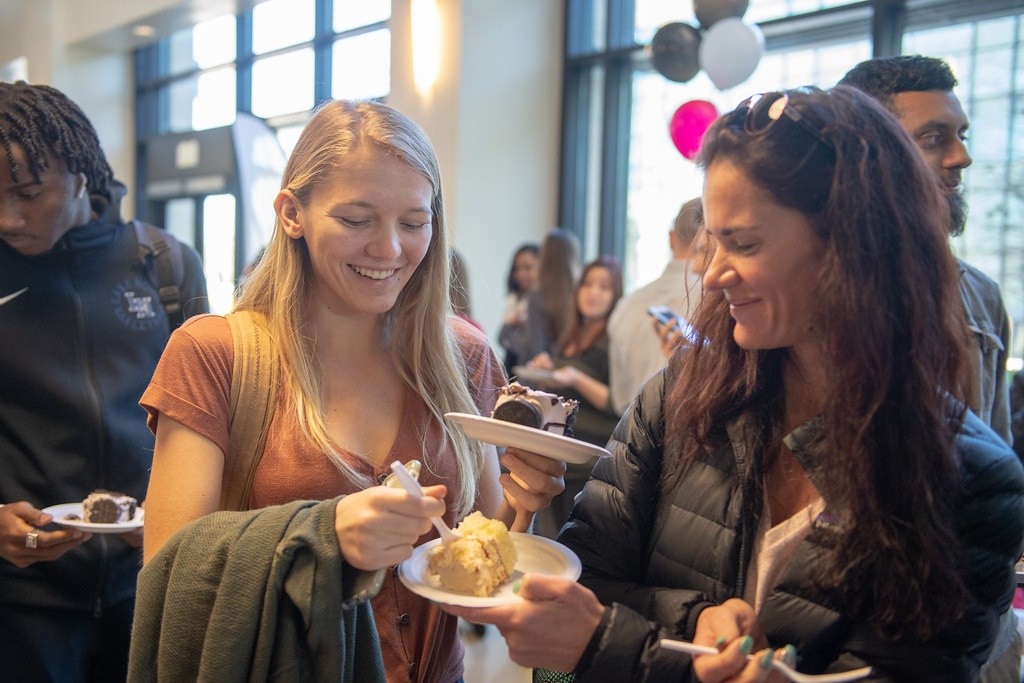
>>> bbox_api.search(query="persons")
[0,77,213,683]
[604,195,703,417]
[494,227,584,396]
[523,256,623,533]
[136,94,567,683]
[831,53,1024,683]
[433,84,1020,683]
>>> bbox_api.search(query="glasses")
[738,94,835,153]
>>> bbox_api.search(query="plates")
[444,412,612,464]
[41,503,145,534]
[398,531,582,608]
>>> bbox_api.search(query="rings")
[26,528,42,549]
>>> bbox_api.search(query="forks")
[659,638,872,683]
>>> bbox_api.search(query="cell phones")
[647,304,710,346]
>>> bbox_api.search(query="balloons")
[670,100,722,163]
[699,17,765,90]
[693,0,749,30]
[649,22,704,84]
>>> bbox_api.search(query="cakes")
[425,510,519,598]
[65,487,139,526]
[490,381,581,441]
